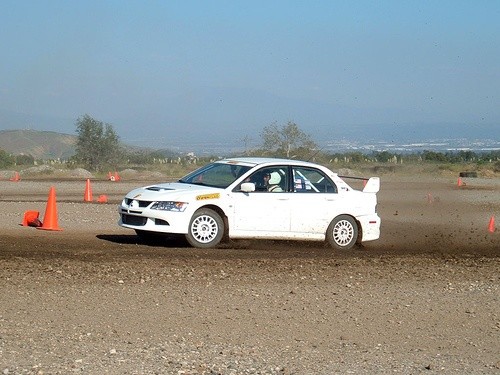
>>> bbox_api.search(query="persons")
[263,171,283,192]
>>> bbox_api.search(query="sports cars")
[117,157,381,251]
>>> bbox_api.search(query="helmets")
[265,169,281,185]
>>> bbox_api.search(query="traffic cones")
[37,186,64,230]
[83,178,94,202]
[363,179,367,187]
[108,169,120,181]
[97,194,107,202]
[23,210,43,227]
[487,215,495,233]
[10,171,20,182]
[458,178,462,187]
[428,191,433,206]
